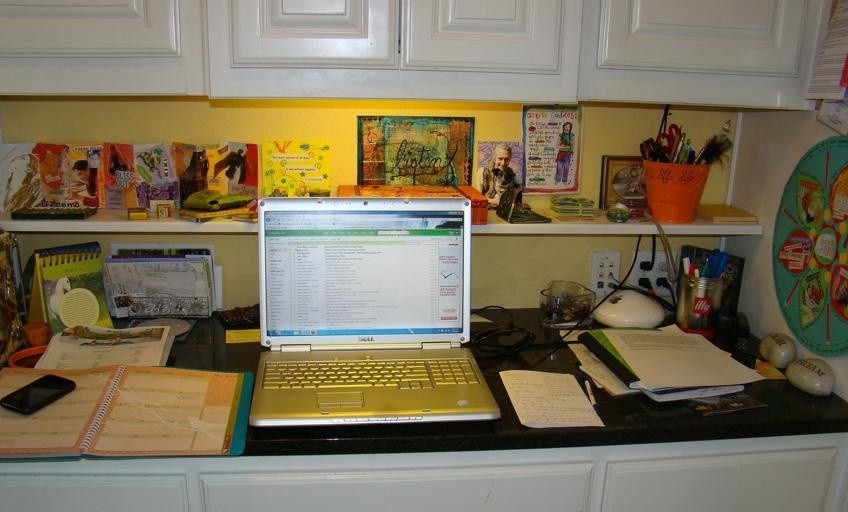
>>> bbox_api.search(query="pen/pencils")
[669,119,733,165]
[676,249,728,330]
[584,376,597,406]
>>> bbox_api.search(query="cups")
[22,322,50,347]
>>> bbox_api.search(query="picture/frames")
[599,154,649,211]
[676,244,745,316]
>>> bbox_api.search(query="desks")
[0,308,848,512]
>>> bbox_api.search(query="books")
[499,370,606,428]
[1,366,253,455]
[803,1,847,136]
[551,195,595,219]
[496,208,551,223]
[0,138,260,225]
[559,325,771,417]
[36,321,175,367]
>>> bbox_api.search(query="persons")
[555,122,575,183]
[479,144,514,208]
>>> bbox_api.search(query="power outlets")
[590,250,621,299]
[630,250,678,297]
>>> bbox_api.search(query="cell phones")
[0,374,76,415]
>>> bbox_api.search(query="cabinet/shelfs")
[206,0,584,105]
[0,1,208,97]
[577,0,835,112]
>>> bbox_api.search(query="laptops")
[248,197,502,427]
